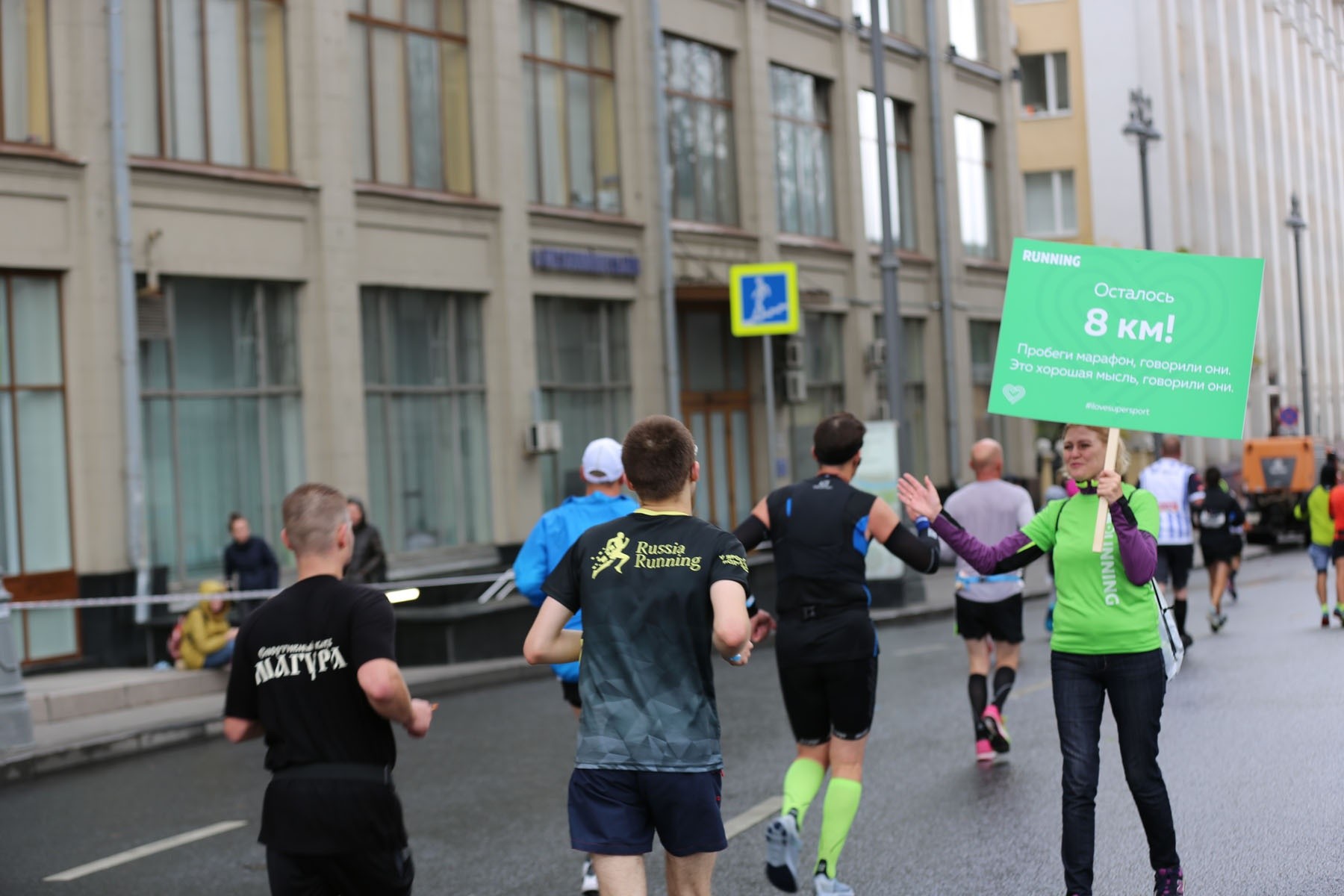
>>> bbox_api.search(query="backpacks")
[166,606,209,659]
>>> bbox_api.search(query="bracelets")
[728,654,742,663]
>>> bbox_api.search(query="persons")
[941,437,1036,764]
[1039,441,1070,633]
[523,415,754,896]
[1294,453,1344,628]
[221,484,434,896]
[342,504,389,587]
[220,513,281,625]
[175,580,239,670]
[513,437,642,896]
[1136,434,1206,650]
[1191,464,1247,634]
[896,424,1184,896]
[733,413,942,896]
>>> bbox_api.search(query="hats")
[582,438,624,484]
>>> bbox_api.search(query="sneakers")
[1153,865,1186,896]
[811,873,854,896]
[764,815,800,894]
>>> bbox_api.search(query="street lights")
[1122,84,1164,250]
[1285,194,1312,436]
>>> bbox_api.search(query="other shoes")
[581,853,599,895]
[1207,607,1229,633]
[1320,606,1330,627]
[982,704,1011,752]
[1043,602,1055,632]
[975,739,996,760]
[1178,633,1193,651]
[1228,584,1237,598]
[1332,605,1344,627]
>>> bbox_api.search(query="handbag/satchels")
[1151,576,1185,678]
